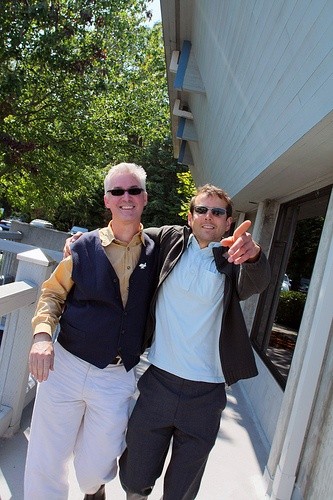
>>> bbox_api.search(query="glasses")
[192,205,226,216]
[106,187,144,195]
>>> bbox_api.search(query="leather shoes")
[85,485,106,500]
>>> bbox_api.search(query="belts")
[108,356,123,366]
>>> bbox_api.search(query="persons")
[23,163,164,500]
[61,186,272,500]
